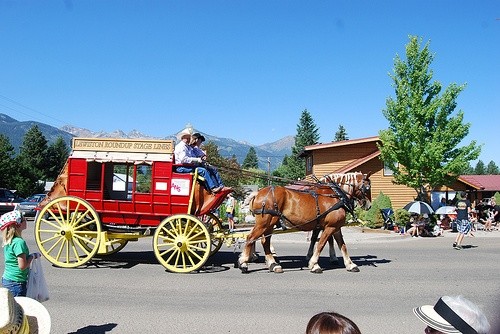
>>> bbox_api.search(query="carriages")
[34,136,372,274]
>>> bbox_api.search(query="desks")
[411,223,424,236]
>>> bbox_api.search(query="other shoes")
[453,242,463,250]
[230,229,234,233]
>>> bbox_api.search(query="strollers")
[380,208,396,230]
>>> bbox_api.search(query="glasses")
[21,218,28,223]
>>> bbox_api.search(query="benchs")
[171,153,195,175]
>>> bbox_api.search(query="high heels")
[211,185,223,196]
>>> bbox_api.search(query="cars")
[14,194,49,220]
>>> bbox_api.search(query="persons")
[453,191,472,250]
[174,129,232,193]
[405,214,436,237]
[0,210,41,297]
[441,215,451,230]
[225,192,236,232]
[213,206,220,220]
[476,199,500,232]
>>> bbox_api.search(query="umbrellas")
[404,201,434,215]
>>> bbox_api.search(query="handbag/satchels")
[27,252,50,303]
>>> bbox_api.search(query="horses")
[236,168,372,275]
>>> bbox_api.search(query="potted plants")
[389,208,410,234]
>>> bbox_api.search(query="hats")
[413,297,478,334]
[177,128,193,140]
[188,133,205,146]
